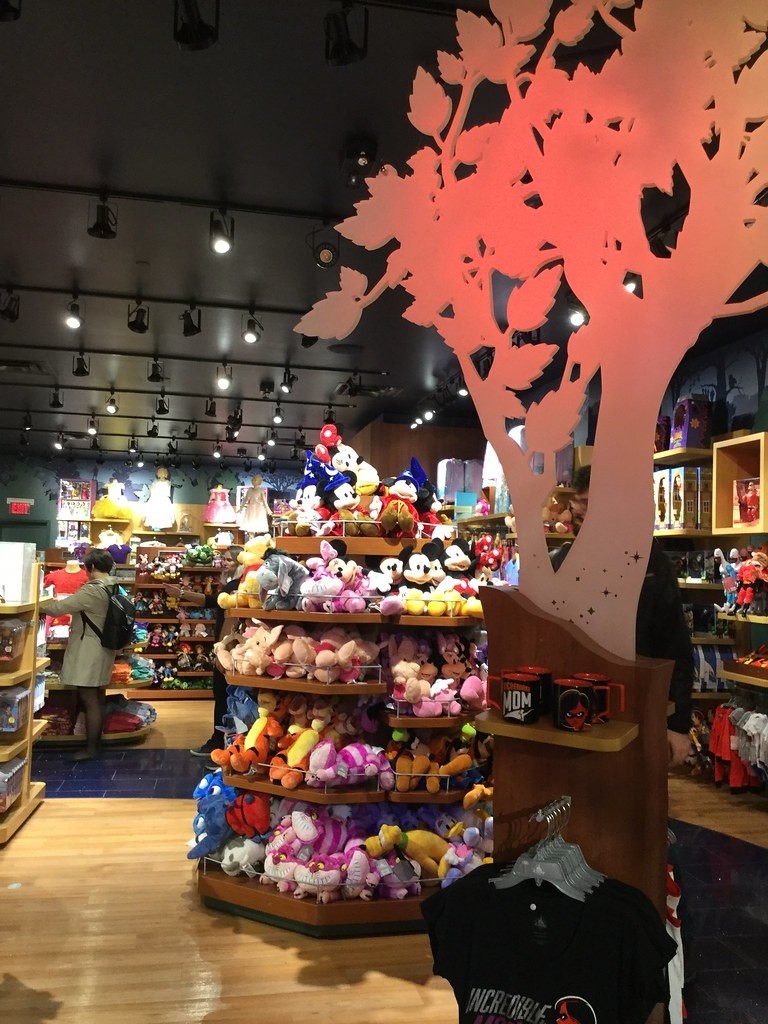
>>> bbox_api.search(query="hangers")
[717,695,768,726]
[485,798,609,904]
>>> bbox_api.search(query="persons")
[202,485,236,523]
[43,549,115,762]
[233,475,273,539]
[98,479,130,519]
[144,467,174,530]
[45,561,90,627]
[222,546,244,583]
[164,574,247,770]
[573,463,692,768]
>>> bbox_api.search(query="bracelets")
[179,590,183,597]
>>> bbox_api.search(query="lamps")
[28,204,376,465]
[409,323,541,429]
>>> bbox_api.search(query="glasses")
[572,497,588,511]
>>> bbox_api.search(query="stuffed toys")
[189,424,510,900]
[133,544,227,689]
[505,495,587,538]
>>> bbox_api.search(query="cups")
[486,665,624,733]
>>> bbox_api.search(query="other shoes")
[63,750,97,761]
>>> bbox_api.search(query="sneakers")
[205,761,222,771]
[190,739,225,756]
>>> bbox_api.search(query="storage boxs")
[651,464,731,690]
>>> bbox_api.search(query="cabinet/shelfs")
[187,520,491,931]
[643,441,734,707]
[52,513,246,698]
[38,622,157,743]
[711,433,768,693]
[0,563,51,846]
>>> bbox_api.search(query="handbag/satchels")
[79,579,137,650]
